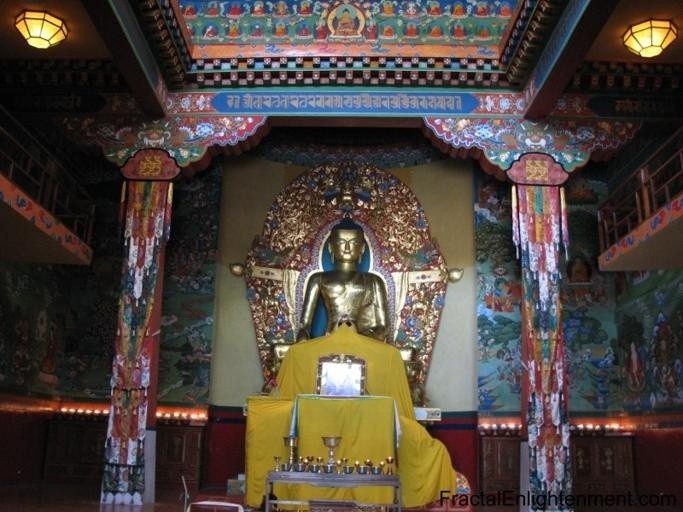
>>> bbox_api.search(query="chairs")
[177,474,244,511]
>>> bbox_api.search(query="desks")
[264,470,401,511]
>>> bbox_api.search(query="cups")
[281,463,381,475]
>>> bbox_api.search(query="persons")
[183,3,511,40]
[272,218,416,361]
